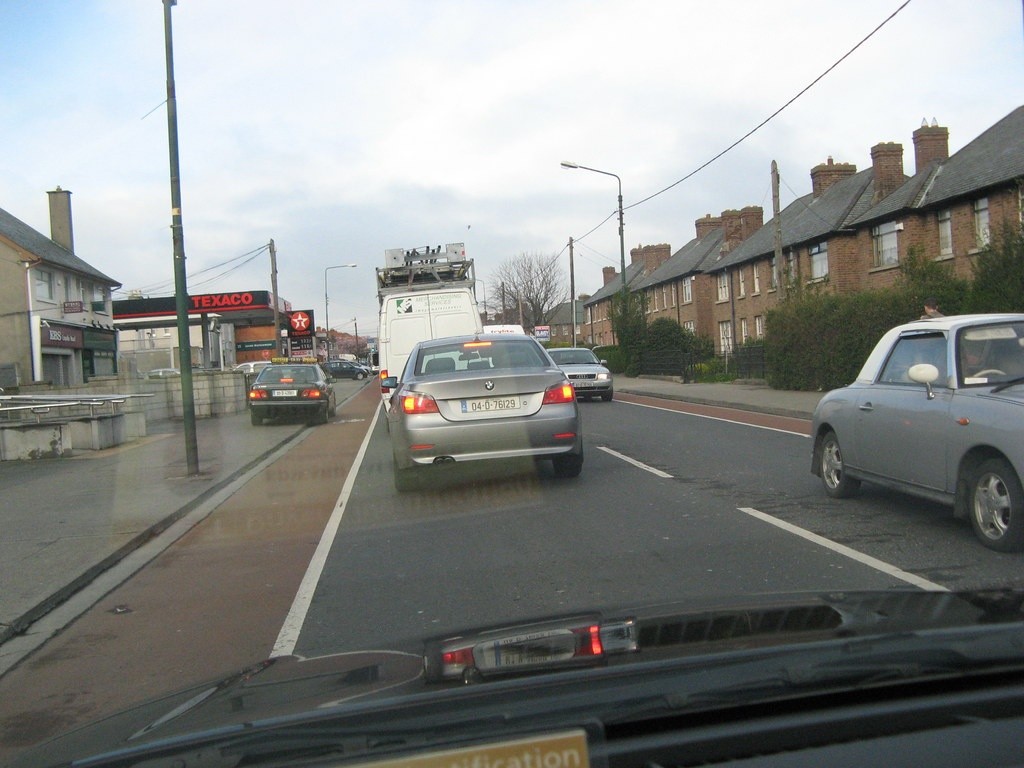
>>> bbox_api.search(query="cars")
[233,361,273,376]
[545,347,613,402]
[147,367,206,376]
[247,357,337,425]
[321,358,370,380]
[810,313,1024,553]
[381,329,584,493]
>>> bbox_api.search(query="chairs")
[269,370,284,382]
[304,369,314,380]
[424,357,455,373]
[493,350,521,368]
[574,353,585,363]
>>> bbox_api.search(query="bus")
[367,349,379,375]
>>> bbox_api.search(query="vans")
[374,241,494,429]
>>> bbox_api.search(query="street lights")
[324,264,358,362]
[561,160,627,314]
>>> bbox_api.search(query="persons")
[920,298,946,320]
